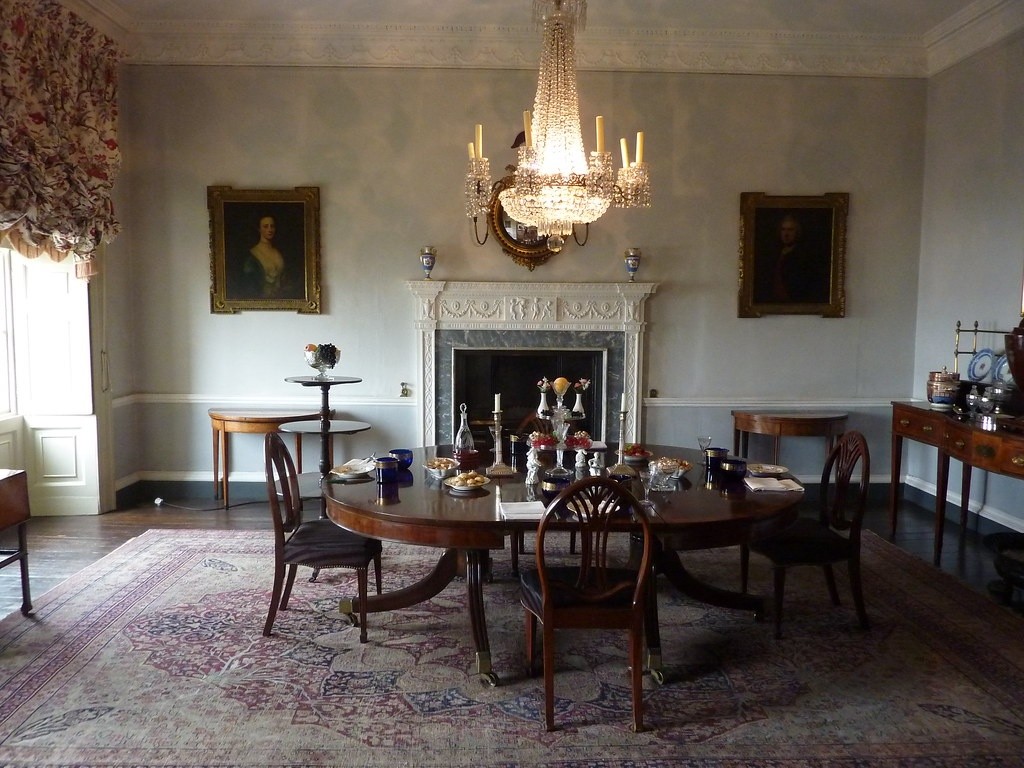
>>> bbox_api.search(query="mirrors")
[490,164,569,270]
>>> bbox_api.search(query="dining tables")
[322,442,765,688]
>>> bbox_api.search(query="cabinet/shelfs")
[277,376,372,520]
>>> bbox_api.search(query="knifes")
[325,479,373,484]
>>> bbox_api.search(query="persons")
[226,212,306,299]
[754,213,823,304]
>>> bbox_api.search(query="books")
[499,501,546,522]
[745,477,786,491]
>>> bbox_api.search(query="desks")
[889,399,1024,568]
[731,408,849,517]
[0,470,33,612]
[210,408,335,505]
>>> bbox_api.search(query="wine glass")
[966,394,982,420]
[489,425,503,453]
[696,435,712,465]
[990,388,1012,414]
[638,470,654,506]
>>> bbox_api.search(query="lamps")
[464,1,650,252]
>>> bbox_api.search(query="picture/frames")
[206,185,322,314]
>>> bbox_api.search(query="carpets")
[736,191,849,319]
[0,527,1024,767]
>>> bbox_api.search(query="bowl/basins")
[540,463,574,501]
[388,449,414,470]
[422,457,461,479]
[648,461,687,479]
[396,469,413,487]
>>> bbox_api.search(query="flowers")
[537,376,554,392]
[571,377,592,394]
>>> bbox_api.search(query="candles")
[494,393,501,411]
[621,392,628,413]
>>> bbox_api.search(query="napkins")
[743,477,805,492]
[500,500,547,520]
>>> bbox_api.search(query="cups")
[978,397,994,416]
[704,447,730,491]
[510,434,523,455]
[376,483,400,506]
[375,457,398,482]
[720,458,747,497]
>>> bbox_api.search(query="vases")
[573,393,584,414]
[538,392,550,414]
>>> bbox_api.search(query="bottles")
[454,403,475,459]
[926,365,961,412]
[967,347,995,382]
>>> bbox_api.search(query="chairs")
[521,478,663,730]
[262,432,382,644]
[740,431,871,639]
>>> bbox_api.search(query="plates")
[444,476,490,490]
[746,463,790,477]
[329,458,377,479]
[615,449,653,465]
[991,353,1015,385]
[564,498,621,519]
